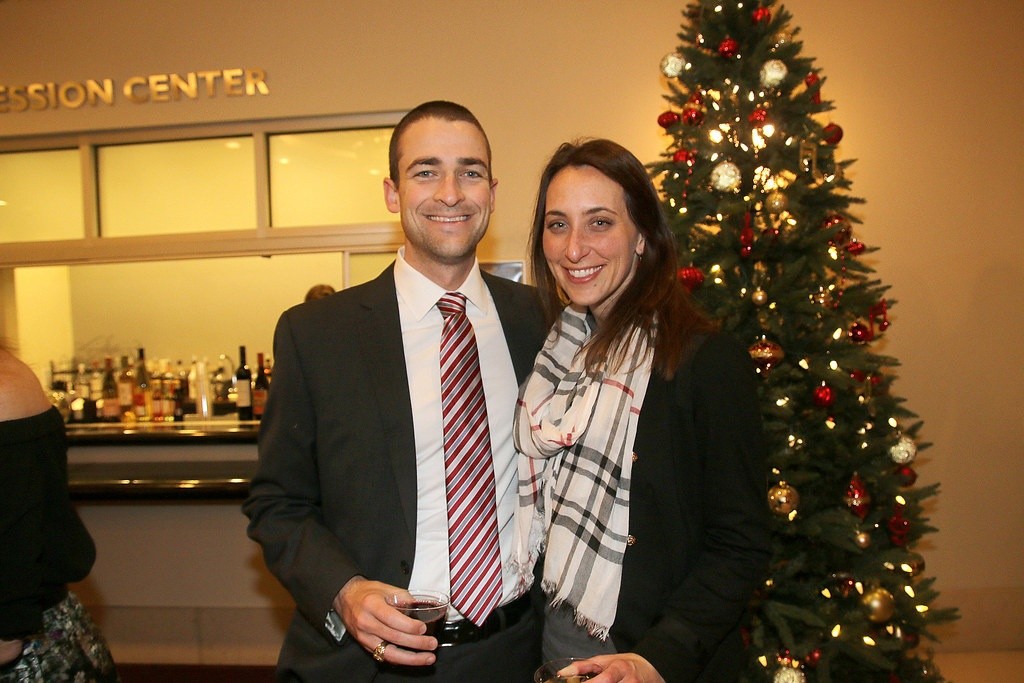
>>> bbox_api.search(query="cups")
[534,657,604,683]
[385,589,450,653]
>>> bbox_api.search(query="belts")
[423,591,538,647]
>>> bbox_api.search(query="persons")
[240,102,565,683]
[0,345,96,683]
[528,140,772,683]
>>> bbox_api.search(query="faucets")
[216,354,237,387]
[190,353,210,419]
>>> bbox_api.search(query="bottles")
[253,352,270,420]
[235,345,252,420]
[264,358,271,384]
[72,348,232,421]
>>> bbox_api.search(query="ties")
[435,292,502,626]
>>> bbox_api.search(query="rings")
[373,640,389,662]
